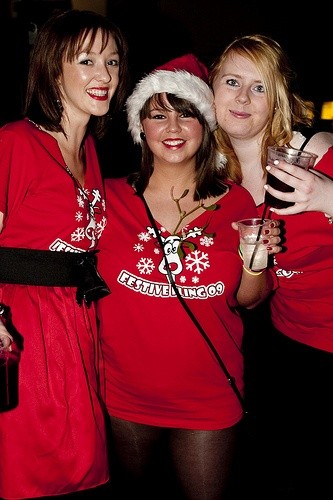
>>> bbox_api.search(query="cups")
[238,218,270,271]
[264,146,319,209]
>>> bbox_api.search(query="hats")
[125,54,218,143]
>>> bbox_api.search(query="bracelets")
[241,263,266,276]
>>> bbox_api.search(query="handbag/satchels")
[0,303,24,414]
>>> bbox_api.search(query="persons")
[71,53,283,500]
[210,33,333,500]
[1,5,129,499]
